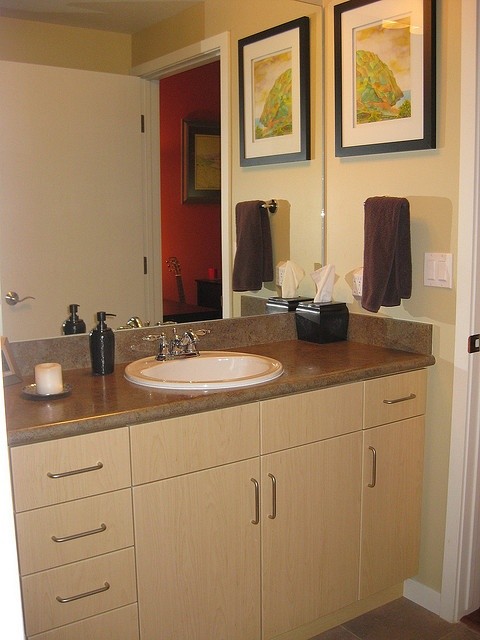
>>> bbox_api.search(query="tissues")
[295,265,350,344]
[262,261,304,312]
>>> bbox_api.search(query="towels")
[231,200,273,293]
[359,197,412,312]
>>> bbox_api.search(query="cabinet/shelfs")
[8,424,140,639]
[128,380,363,640]
[359,368,427,604]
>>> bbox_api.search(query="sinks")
[123,346,285,390]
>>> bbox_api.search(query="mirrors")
[0,0,324,344]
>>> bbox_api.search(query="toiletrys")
[63,304,86,335]
[90,312,116,374]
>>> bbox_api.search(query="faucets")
[125,316,150,328]
[172,327,200,358]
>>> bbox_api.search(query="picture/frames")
[179,119,221,205]
[0,336,24,387]
[237,15,310,167]
[333,1,436,157]
